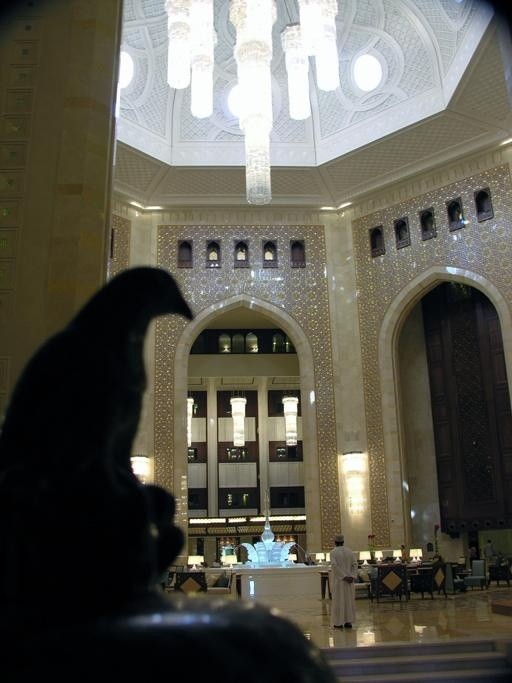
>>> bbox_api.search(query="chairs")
[318,560,512,603]
[166,571,236,599]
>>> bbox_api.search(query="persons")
[465,539,493,569]
[330,534,358,628]
[399,545,409,560]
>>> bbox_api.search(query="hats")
[334,534,344,542]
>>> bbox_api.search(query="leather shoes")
[334,622,352,628]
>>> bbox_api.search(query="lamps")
[188,555,204,570]
[187,398,194,447]
[130,456,149,484]
[393,550,403,562]
[281,394,299,446]
[375,551,384,561]
[230,396,247,447]
[166,1,340,205]
[288,554,297,564]
[359,551,372,565]
[222,555,237,569]
[316,553,331,563]
[410,549,423,561]
